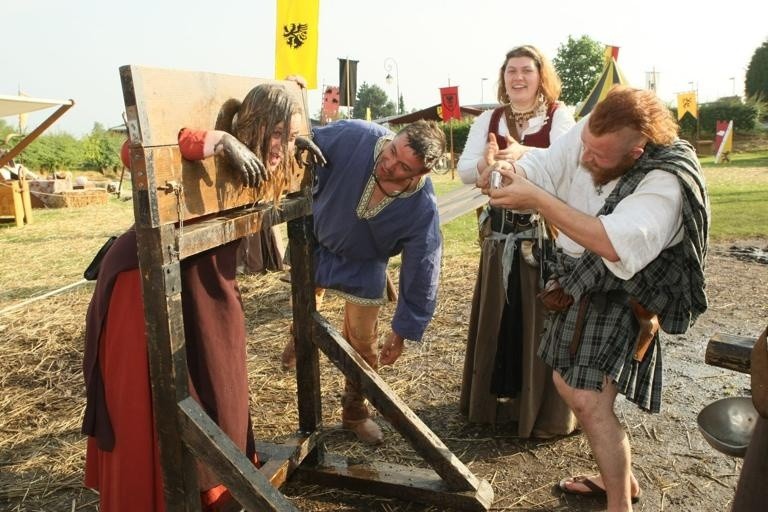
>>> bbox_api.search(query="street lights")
[386,63,400,115]
[480,75,490,110]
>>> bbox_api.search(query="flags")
[714,116,734,165]
[337,57,359,108]
[440,86,463,122]
[675,90,699,123]
[274,1,319,90]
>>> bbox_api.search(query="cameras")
[491,171,502,192]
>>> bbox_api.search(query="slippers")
[558,476,643,503]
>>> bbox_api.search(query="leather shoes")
[343,404,383,445]
[282,322,296,369]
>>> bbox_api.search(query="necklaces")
[371,147,415,198]
[594,181,608,196]
[506,87,544,128]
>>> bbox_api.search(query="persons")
[474,81,712,511]
[454,44,577,440]
[79,81,330,512]
[277,116,448,448]
[729,323,767,511]
